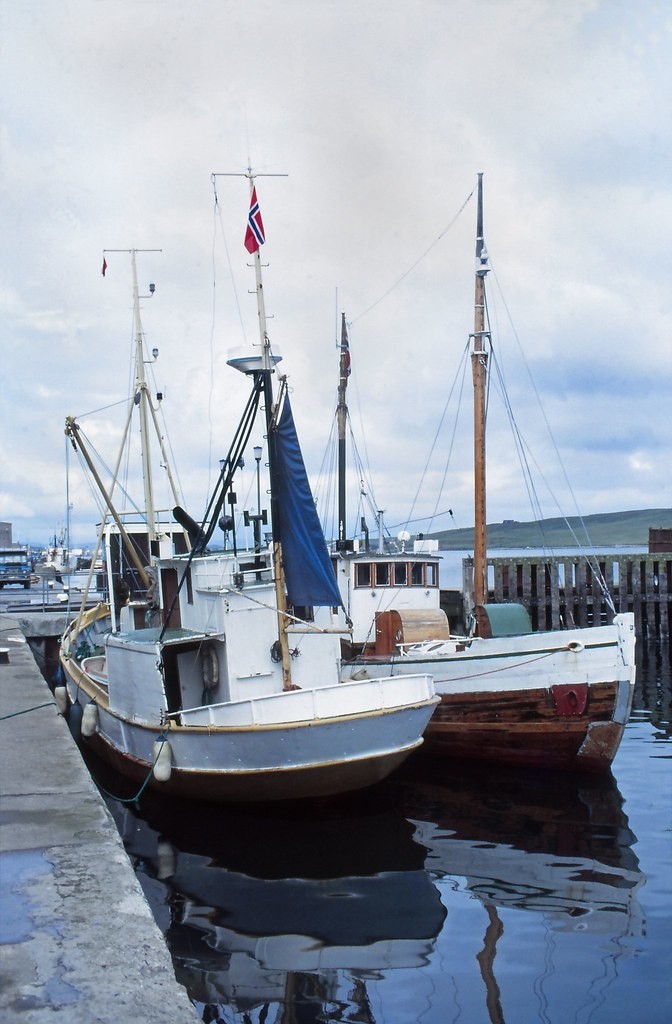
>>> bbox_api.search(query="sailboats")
[33,153,635,806]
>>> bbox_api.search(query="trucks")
[0,545,31,591]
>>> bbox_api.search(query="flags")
[244,184,265,255]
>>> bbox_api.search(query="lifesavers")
[202,647,219,689]
